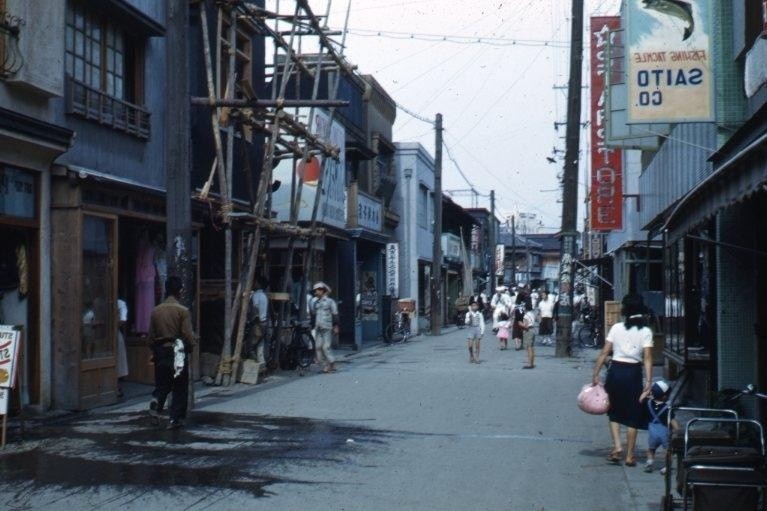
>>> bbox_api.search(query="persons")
[455,284,559,368]
[150,276,196,428]
[639,381,679,474]
[580,297,591,312]
[84,299,129,397]
[308,282,339,373]
[245,275,270,364]
[593,293,656,465]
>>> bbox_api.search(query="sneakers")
[168,418,187,428]
[660,467,668,474]
[624,455,638,466]
[318,367,336,374]
[607,450,624,463]
[542,338,553,345]
[500,345,524,351]
[643,460,655,472]
[149,399,160,417]
[523,364,535,371]
[468,360,481,365]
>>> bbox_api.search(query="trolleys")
[682,416,767,509]
[661,404,741,511]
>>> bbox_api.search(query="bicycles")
[243,318,316,371]
[455,310,468,329]
[577,308,603,349]
[384,312,411,345]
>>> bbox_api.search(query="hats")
[651,381,671,396]
[310,283,327,290]
